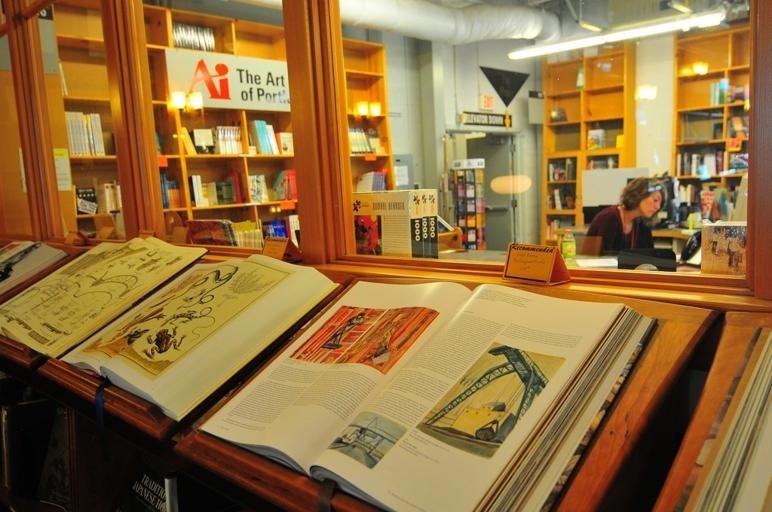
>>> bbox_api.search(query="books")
[247,119,293,155]
[1,236,210,360]
[185,214,299,249]
[64,112,105,157]
[181,126,243,155]
[159,172,181,208]
[76,183,122,215]
[545,76,751,244]
[357,172,384,192]
[248,168,298,202]
[671,323,771,511]
[349,126,381,154]
[189,172,243,206]
[58,252,343,425]
[199,280,661,511]
[352,188,439,259]
[1,239,69,294]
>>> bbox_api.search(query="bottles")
[680,201,703,231]
[561,222,576,269]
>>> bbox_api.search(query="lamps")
[508,0,726,60]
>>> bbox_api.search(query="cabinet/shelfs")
[341,36,396,251]
[114,1,301,252]
[673,21,750,229]
[1,0,141,252]
[540,42,635,248]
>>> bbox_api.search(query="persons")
[578,176,665,258]
